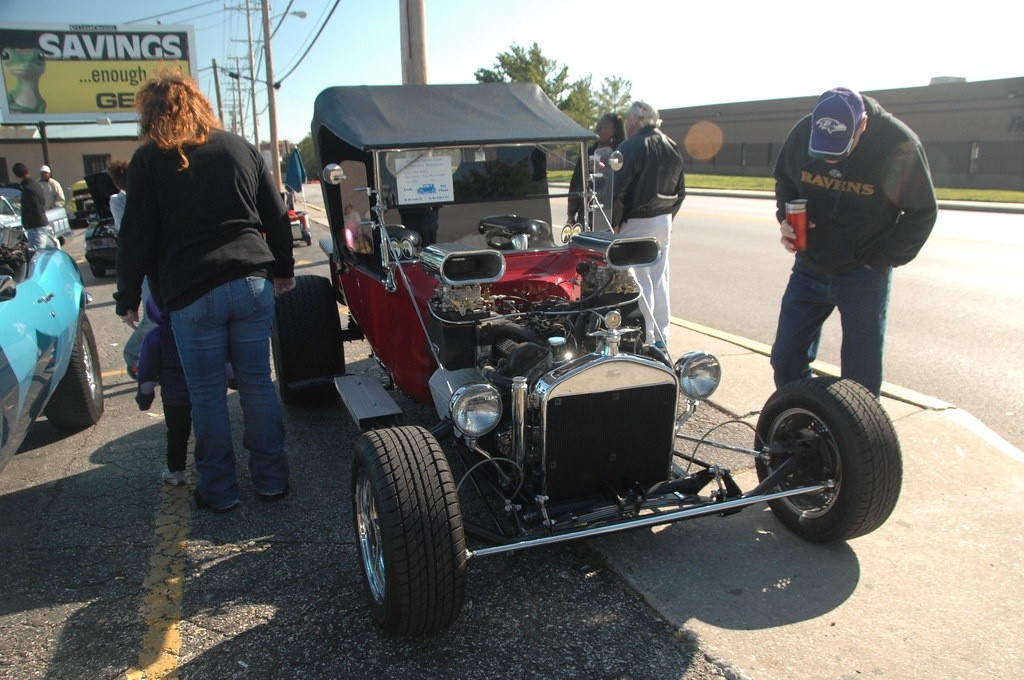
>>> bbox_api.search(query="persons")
[772,88,938,404]
[112,73,294,509]
[12,163,48,249]
[605,102,685,352]
[134,295,191,485]
[108,160,150,301]
[567,113,626,233]
[35,165,65,212]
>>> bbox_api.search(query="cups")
[785,201,807,251]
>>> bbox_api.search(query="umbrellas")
[285,145,308,212]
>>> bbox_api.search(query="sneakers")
[195,490,244,513]
[161,470,187,488]
[250,472,290,499]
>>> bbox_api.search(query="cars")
[0,191,105,472]
[272,82,905,640]
[83,151,125,279]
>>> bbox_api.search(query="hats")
[807,87,867,164]
[40,165,51,174]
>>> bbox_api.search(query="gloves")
[135,391,155,411]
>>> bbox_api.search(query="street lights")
[262,11,307,199]
